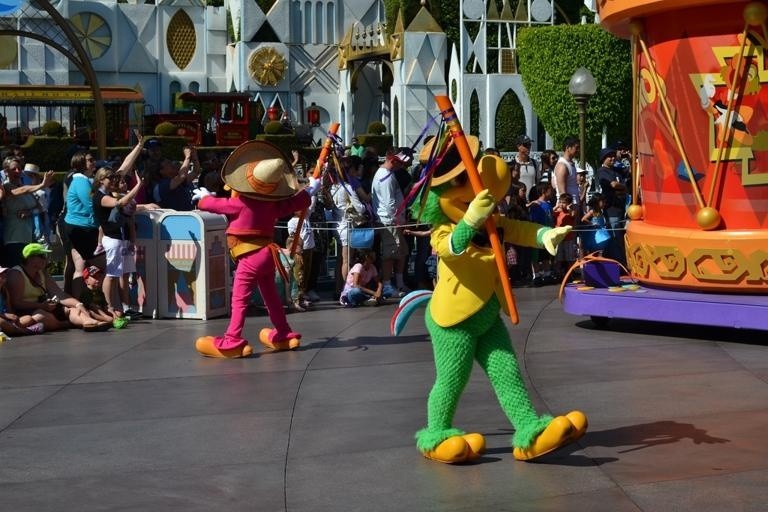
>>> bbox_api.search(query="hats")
[0,266,8,274]
[598,147,617,163]
[22,243,52,259]
[83,265,104,279]
[22,163,43,177]
[515,134,534,145]
[385,149,411,164]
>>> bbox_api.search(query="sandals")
[113,318,129,328]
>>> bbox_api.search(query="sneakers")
[123,309,143,319]
[382,284,412,299]
[26,321,45,335]
[362,299,378,306]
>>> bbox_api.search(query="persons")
[478,138,631,288]
[286,136,447,310]
[1,128,202,336]
[191,140,321,359]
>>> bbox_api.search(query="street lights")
[568,66,599,182]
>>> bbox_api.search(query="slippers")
[83,320,111,333]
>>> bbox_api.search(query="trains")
[1,82,314,178]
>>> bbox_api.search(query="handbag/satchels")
[344,201,373,228]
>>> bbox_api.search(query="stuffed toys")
[389,134,588,464]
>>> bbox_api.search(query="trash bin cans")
[574,224,621,287]
[118,209,230,321]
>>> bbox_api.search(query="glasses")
[104,174,117,182]
[85,158,96,163]
